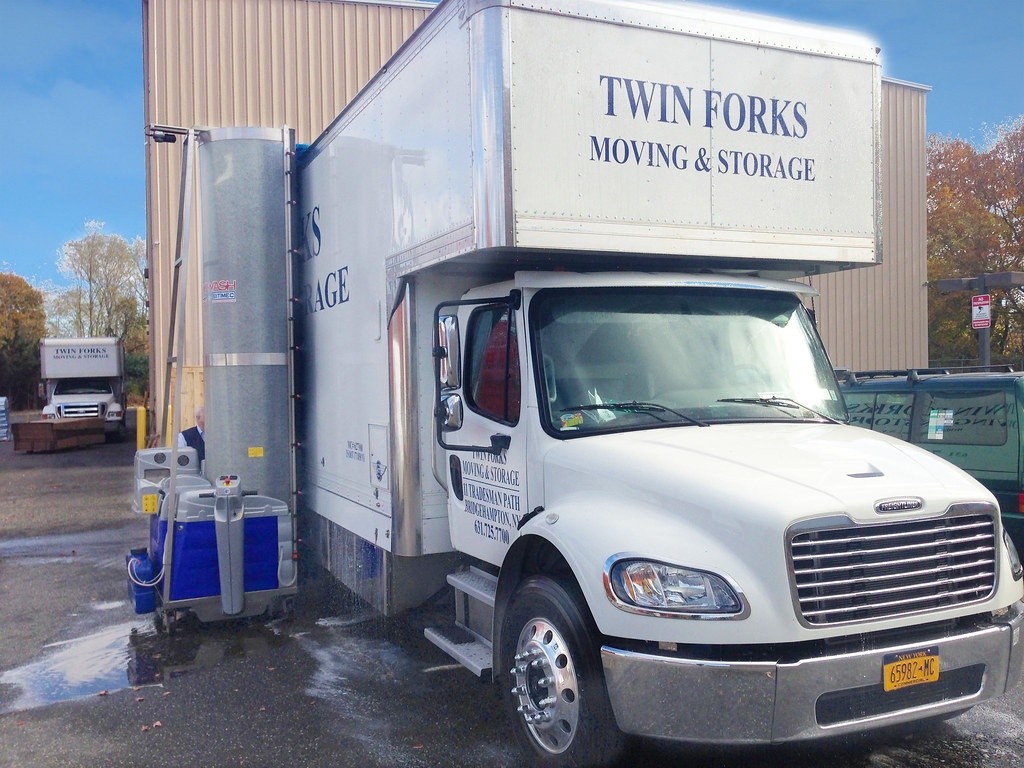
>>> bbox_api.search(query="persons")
[178,406,205,472]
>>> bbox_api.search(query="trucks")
[267,0,1024,768]
[38,337,129,443]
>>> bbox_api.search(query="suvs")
[834,365,1024,561]
